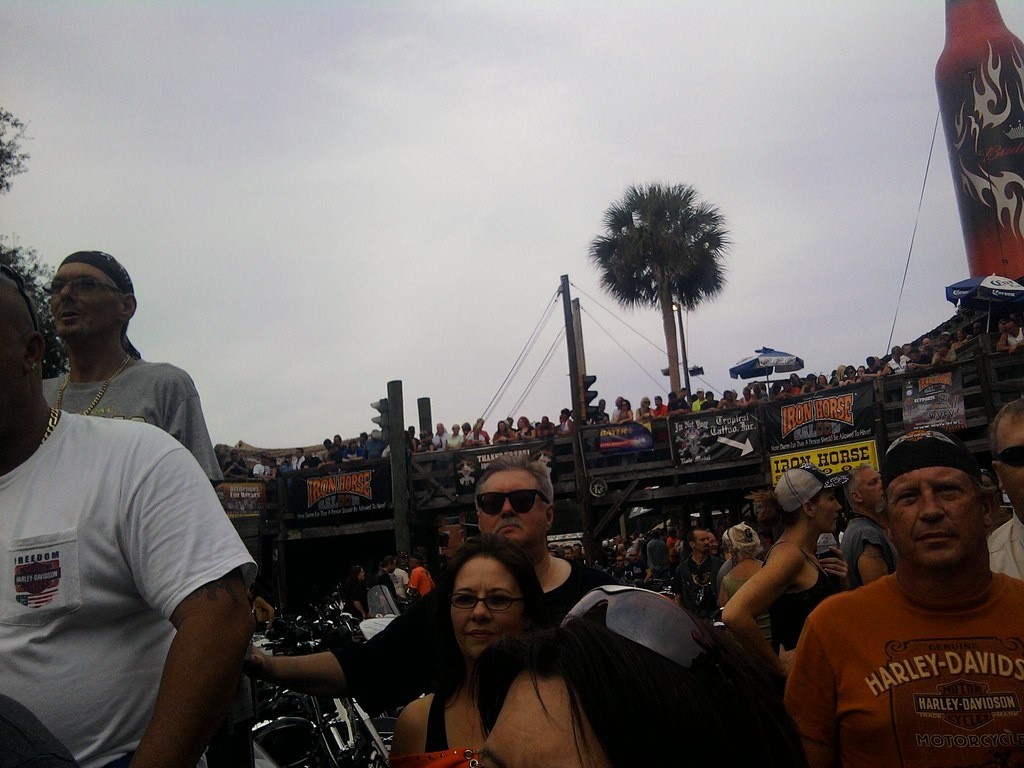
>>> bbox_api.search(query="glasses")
[559,584,720,669]
[449,593,525,612]
[992,446,1024,467]
[476,489,549,515]
[41,278,126,297]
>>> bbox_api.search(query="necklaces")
[56,354,131,415]
[38,408,59,449]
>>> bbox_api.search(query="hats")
[410,552,424,561]
[934,341,949,348]
[775,462,850,513]
[881,428,981,493]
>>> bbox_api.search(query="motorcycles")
[243,579,402,768]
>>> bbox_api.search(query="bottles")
[815,531,837,561]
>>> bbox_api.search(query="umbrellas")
[728,347,804,397]
[946,272,1024,334]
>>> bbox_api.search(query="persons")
[214,311,1024,768]
[40,250,225,490]
[0,268,261,768]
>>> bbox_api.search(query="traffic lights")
[370,398,391,447]
[576,372,601,422]
